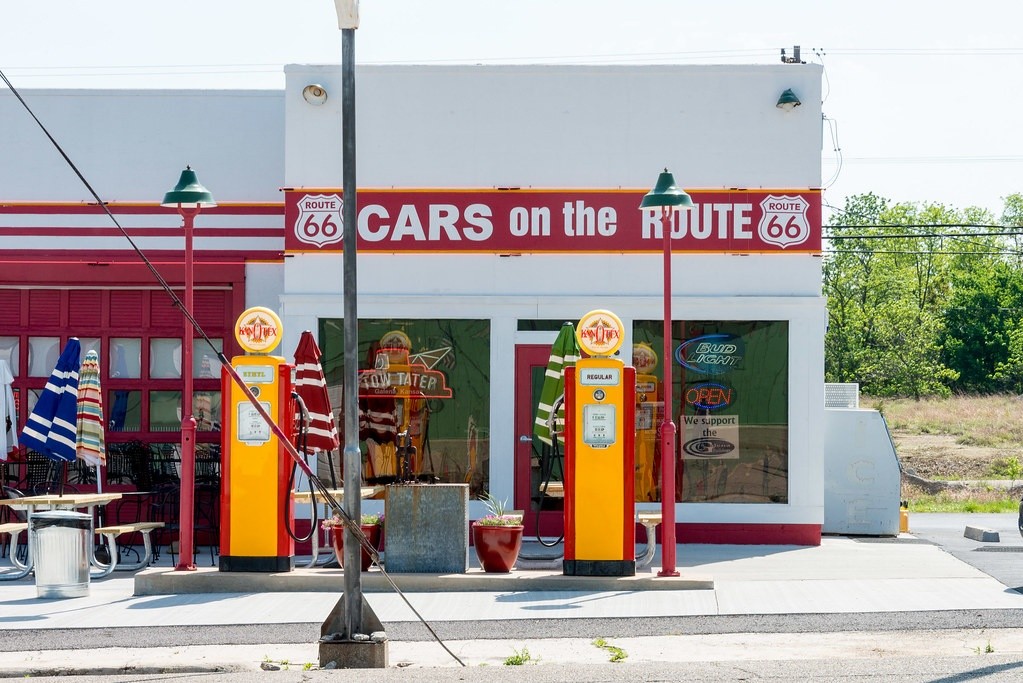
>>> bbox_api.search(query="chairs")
[0,460,221,571]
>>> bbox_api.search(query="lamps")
[776,89,801,111]
[304,84,328,105]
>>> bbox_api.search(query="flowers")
[472,490,523,526]
[321,511,385,530]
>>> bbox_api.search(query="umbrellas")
[195,355,211,450]
[109,344,130,432]
[359,342,401,444]
[0,359,19,461]
[294,330,340,457]
[534,322,582,448]
[76,350,106,493]
[20,337,80,464]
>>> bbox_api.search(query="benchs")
[90,522,165,578]
[636,510,662,569]
[0,522,27,580]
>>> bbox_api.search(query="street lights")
[162,167,216,569]
[638,169,692,575]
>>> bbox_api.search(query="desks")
[117,491,164,556]
[539,480,564,498]
[292,485,385,568]
[0,493,122,579]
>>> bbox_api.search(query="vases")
[331,524,381,570]
[472,525,525,573]
